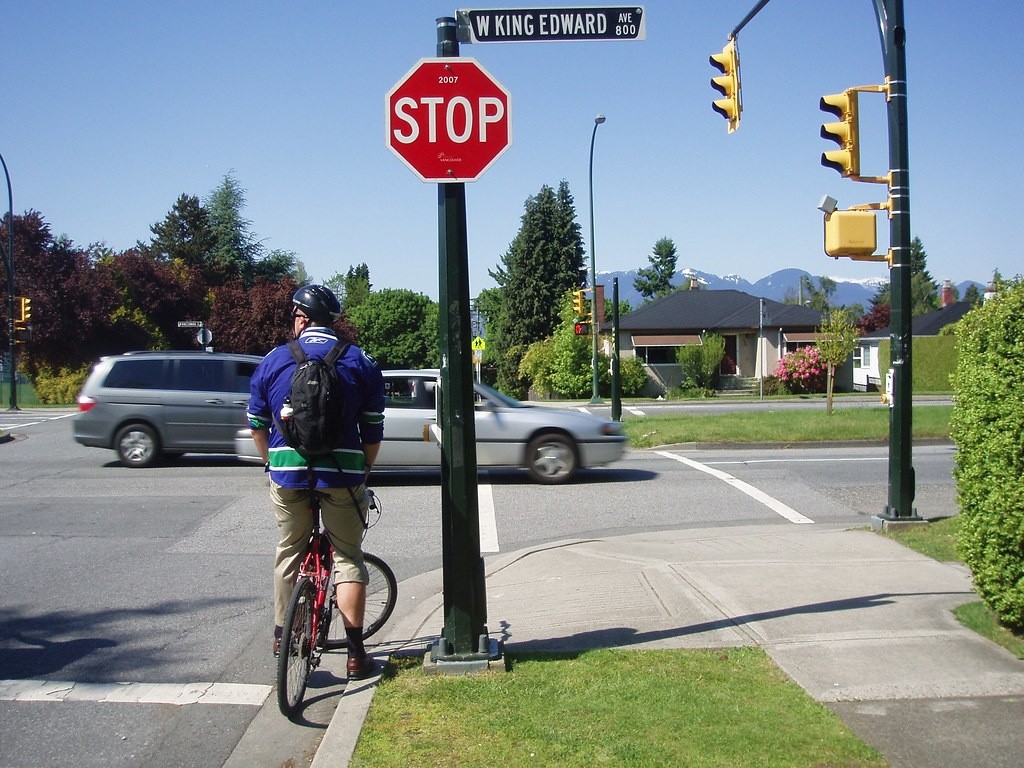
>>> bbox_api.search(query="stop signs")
[384,55,514,183]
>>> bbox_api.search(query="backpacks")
[287,339,352,462]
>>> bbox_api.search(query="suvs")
[70,349,266,469]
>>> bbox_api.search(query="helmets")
[292,285,343,325]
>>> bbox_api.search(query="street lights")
[588,114,606,404]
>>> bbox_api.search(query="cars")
[231,369,629,486]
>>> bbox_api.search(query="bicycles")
[274,461,399,717]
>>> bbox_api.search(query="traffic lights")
[575,323,589,335]
[572,290,584,314]
[820,89,861,180]
[21,297,32,322]
[709,35,741,135]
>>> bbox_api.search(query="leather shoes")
[347,657,373,679]
[273,639,294,656]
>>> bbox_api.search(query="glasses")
[290,311,308,319]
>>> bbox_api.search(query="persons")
[247,284,386,681]
